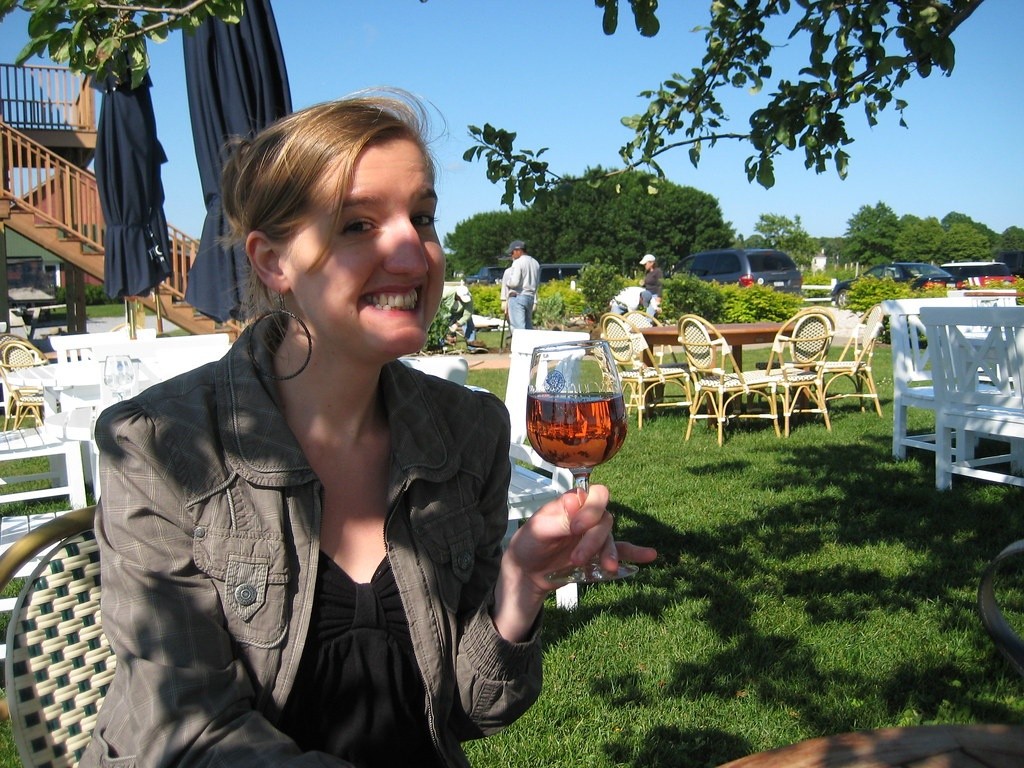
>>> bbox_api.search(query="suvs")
[665,247,804,296]
[465,266,508,284]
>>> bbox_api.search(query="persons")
[437,286,476,352]
[609,254,663,326]
[77,103,657,768]
[501,240,542,358]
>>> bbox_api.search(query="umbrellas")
[182,1,293,343]
[93,0,174,337]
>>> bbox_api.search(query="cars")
[939,261,1019,291]
[831,261,956,307]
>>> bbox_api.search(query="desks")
[629,321,796,421]
[45,402,115,504]
[955,331,1024,474]
[7,360,100,417]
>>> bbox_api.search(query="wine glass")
[526,341,640,586]
[103,354,136,401]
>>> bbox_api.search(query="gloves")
[446,324,457,335]
[532,304,537,313]
[501,301,508,311]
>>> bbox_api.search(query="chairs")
[98,328,157,359]
[0,333,53,419]
[502,326,589,613]
[0,340,52,433]
[819,302,885,422]
[398,356,469,388]
[742,306,838,439]
[0,426,87,509]
[676,314,781,447]
[0,501,117,768]
[600,313,693,433]
[48,333,100,364]
[623,311,690,414]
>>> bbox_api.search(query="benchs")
[920,306,1024,492]
[882,297,1017,473]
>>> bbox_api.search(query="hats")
[639,254,656,265]
[505,241,526,254]
[457,285,471,303]
[641,290,652,308]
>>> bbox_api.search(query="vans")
[538,263,599,283]
